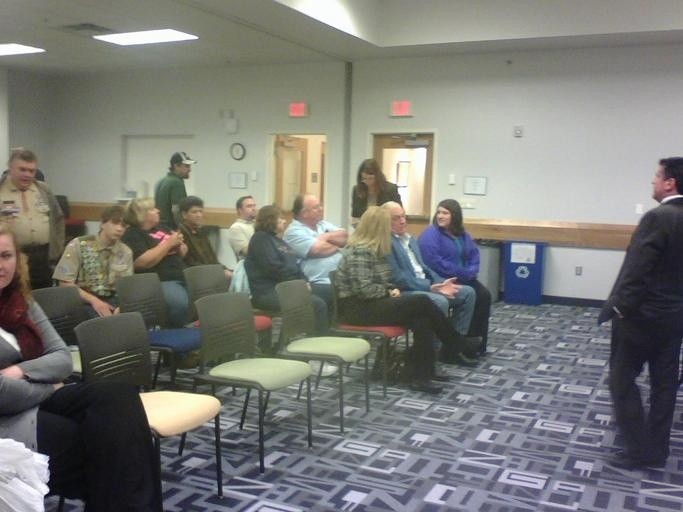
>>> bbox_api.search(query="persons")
[417,199,491,356]
[333,206,482,393]
[0,223,162,511]
[52,206,134,318]
[283,193,349,319]
[597,157,683,469]
[153,152,197,231]
[351,159,402,229]
[121,197,224,369]
[381,201,478,366]
[175,196,233,292]
[0,147,45,182]
[244,206,339,378]
[0,151,66,290]
[228,196,257,261]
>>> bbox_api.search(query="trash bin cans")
[503,239,547,306]
[474,237,503,302]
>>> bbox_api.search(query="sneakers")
[308,360,339,377]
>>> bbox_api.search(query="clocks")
[229,142,246,161]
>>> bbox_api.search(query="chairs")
[114,272,203,391]
[194,293,313,473]
[73,311,223,501]
[29,286,89,382]
[328,270,411,400]
[274,279,371,433]
[182,264,273,332]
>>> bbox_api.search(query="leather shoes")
[641,455,666,468]
[465,335,484,350]
[428,365,448,381]
[411,378,443,394]
[608,451,641,468]
[445,351,479,366]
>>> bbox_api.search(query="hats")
[171,152,197,165]
